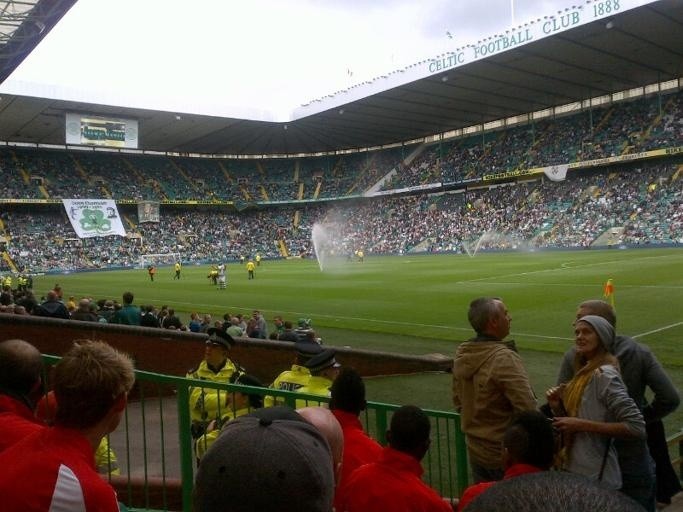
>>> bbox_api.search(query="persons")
[1,91,682,272]
[449,298,682,511]
[0,255,454,511]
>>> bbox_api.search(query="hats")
[576,315,614,352]
[195,404,335,511]
[292,340,321,360]
[204,328,236,352]
[303,349,341,373]
[228,371,263,408]
